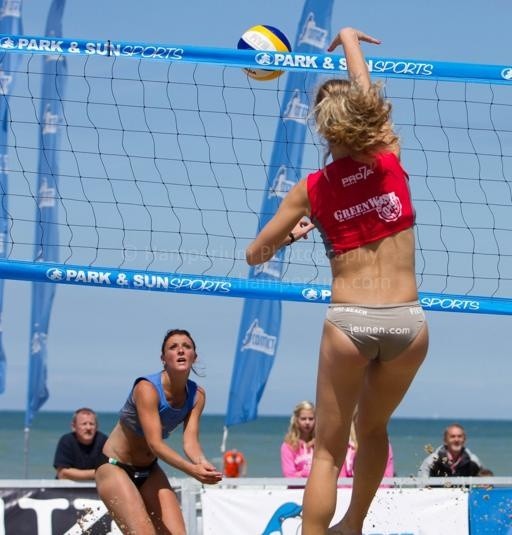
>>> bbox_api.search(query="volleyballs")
[237,25,291,80]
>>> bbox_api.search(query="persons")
[95,329,222,535]
[223,447,246,477]
[479,469,494,488]
[53,409,109,480]
[247,27,429,535]
[280,400,348,489]
[417,425,483,489]
[337,404,394,489]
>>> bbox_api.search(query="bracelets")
[286,232,295,246]
